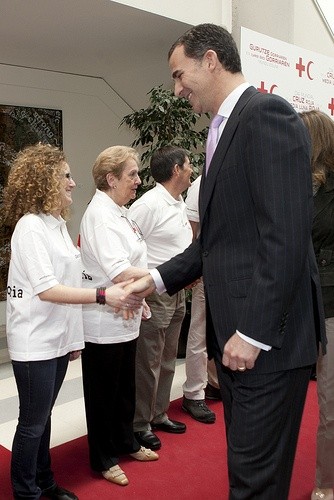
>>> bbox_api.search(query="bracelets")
[96,286,106,306]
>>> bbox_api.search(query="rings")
[126,303,129,307]
[238,366,246,370]
[192,283,196,287]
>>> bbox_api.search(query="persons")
[114,23,328,500]
[182,174,222,423]
[80,145,202,485]
[296,110,334,500]
[3,145,143,500]
[128,146,194,449]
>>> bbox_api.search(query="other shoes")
[101,464,129,485]
[181,394,216,423]
[44,484,78,500]
[129,444,159,461]
[204,383,222,400]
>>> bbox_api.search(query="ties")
[205,113,224,177]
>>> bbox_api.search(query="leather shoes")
[151,418,186,434]
[134,429,161,450]
